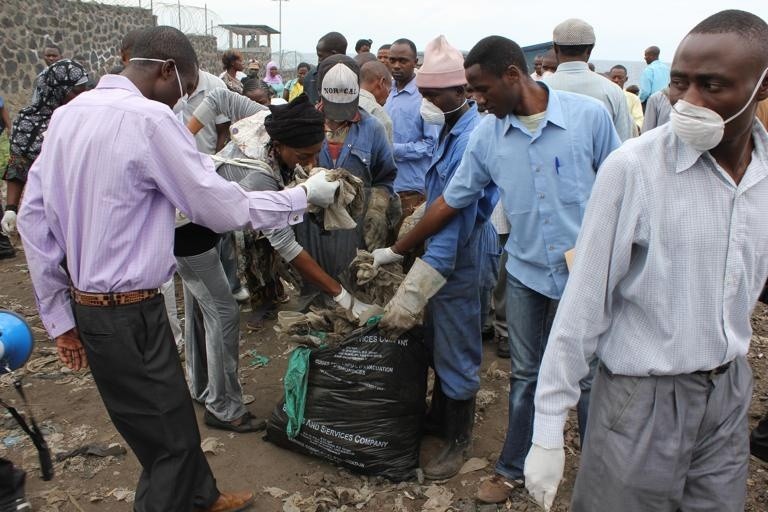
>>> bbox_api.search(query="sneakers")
[204,407,267,432]
[477,472,525,503]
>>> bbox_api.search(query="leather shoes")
[232,287,249,300]
[195,490,254,512]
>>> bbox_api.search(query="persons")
[0,9,768,511]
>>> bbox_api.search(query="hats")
[414,33,468,88]
[553,17,595,45]
[317,54,360,121]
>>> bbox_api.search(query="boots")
[423,374,475,479]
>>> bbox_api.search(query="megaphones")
[0,310,34,378]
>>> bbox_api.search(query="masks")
[173,67,189,114]
[669,66,767,152]
[420,97,465,125]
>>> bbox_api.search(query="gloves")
[296,171,447,340]
[0,211,16,238]
[523,445,565,512]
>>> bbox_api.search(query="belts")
[69,279,159,306]
[690,363,731,376]
